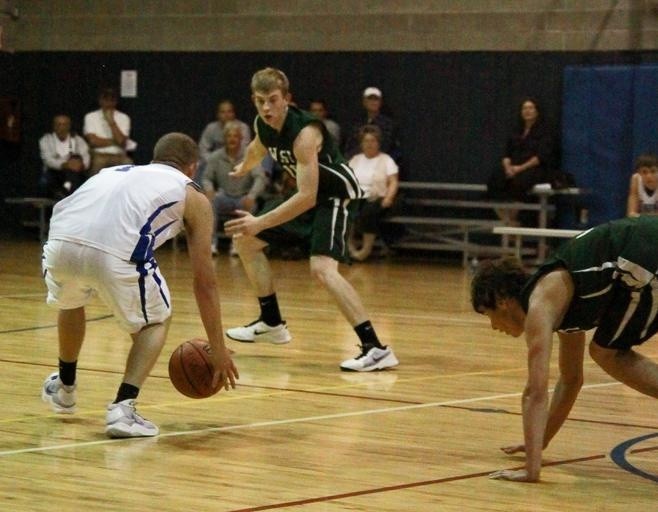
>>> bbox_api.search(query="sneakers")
[229,244,237,256]
[42,371,76,413]
[340,342,399,371]
[225,317,292,345]
[211,243,219,256]
[105,399,159,436]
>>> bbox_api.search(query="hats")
[363,87,382,98]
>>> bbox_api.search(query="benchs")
[6,197,61,240]
[399,179,594,267]
[383,216,522,258]
[492,226,585,264]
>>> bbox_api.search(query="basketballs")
[167,338,223,399]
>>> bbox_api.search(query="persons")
[224,67,399,371]
[42,132,239,439]
[487,98,556,226]
[82,85,136,176]
[627,154,658,217]
[345,126,399,260]
[39,117,91,201]
[471,216,658,482]
[198,101,250,153]
[201,122,267,255]
[310,100,339,149]
[346,88,395,157]
[254,177,315,257]
